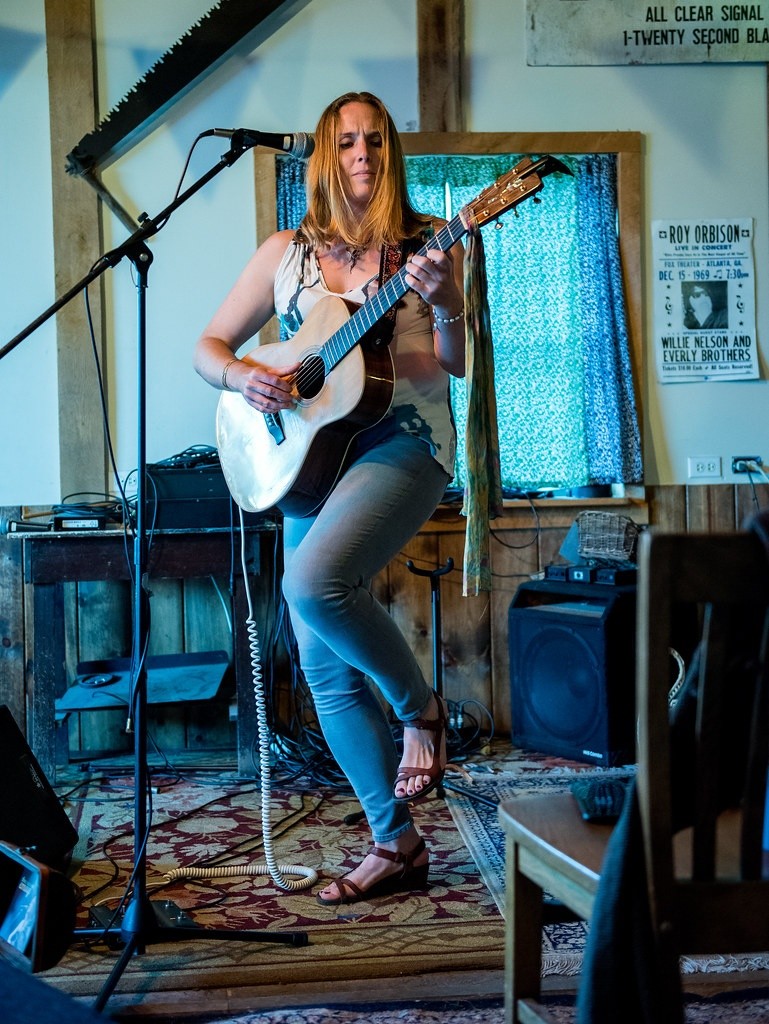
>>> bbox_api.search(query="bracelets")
[432,306,464,324]
[221,359,239,389]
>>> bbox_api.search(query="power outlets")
[732,456,761,473]
[687,457,721,477]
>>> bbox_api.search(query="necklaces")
[341,237,372,274]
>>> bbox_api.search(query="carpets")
[446,763,769,977]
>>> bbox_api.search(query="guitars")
[216,156,550,519]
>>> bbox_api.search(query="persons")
[683,282,729,329]
[192,92,465,906]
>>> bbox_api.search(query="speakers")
[0,702,81,868]
[509,575,638,771]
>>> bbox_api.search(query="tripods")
[1,150,308,1015]
[338,554,500,825]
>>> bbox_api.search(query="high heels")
[392,690,448,804]
[315,837,430,906]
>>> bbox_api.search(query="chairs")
[499,531,769,1024]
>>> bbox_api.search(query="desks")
[4,524,282,779]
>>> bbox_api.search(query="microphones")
[208,129,318,160]
